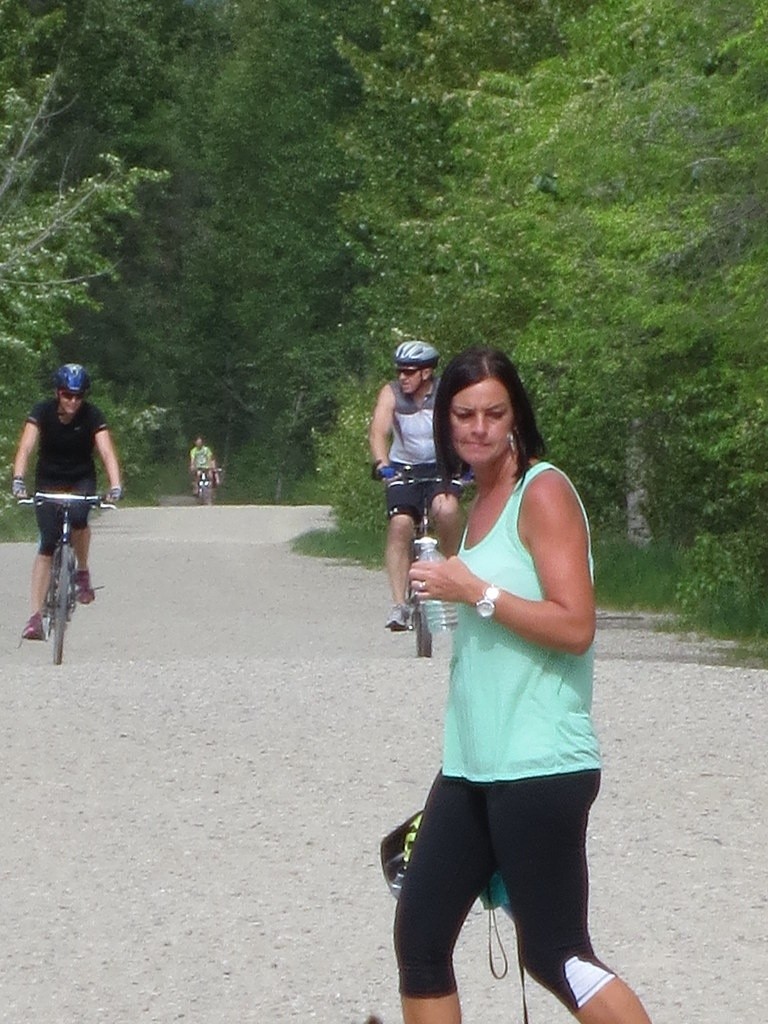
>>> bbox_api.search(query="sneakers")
[385,603,410,629]
[75,567,92,603]
[21,613,45,640]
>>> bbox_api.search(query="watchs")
[475,583,504,620]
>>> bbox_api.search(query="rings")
[418,580,425,590]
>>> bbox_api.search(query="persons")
[391,343,652,1024]
[370,340,474,631]
[14,361,120,643]
[192,438,220,499]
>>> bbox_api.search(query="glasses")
[395,367,424,375]
[60,392,84,399]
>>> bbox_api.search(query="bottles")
[414,534,460,636]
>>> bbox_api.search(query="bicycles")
[14,489,122,666]
[381,469,476,659]
[192,466,222,506]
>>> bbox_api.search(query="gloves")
[378,466,395,479]
[463,468,474,481]
[106,486,122,502]
[12,476,29,499]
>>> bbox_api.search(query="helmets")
[394,340,440,369]
[55,364,90,394]
